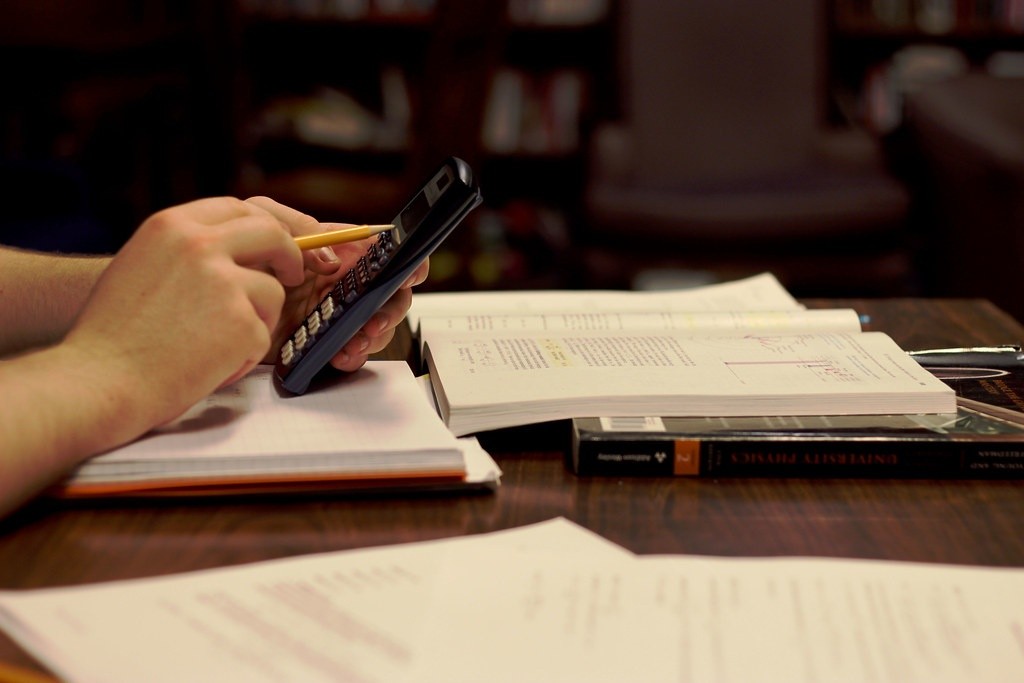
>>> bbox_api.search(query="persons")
[0,193,434,530]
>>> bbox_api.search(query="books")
[32,354,461,504]
[566,340,1023,479]
[396,269,961,441]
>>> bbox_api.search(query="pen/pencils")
[295,224,396,249]
